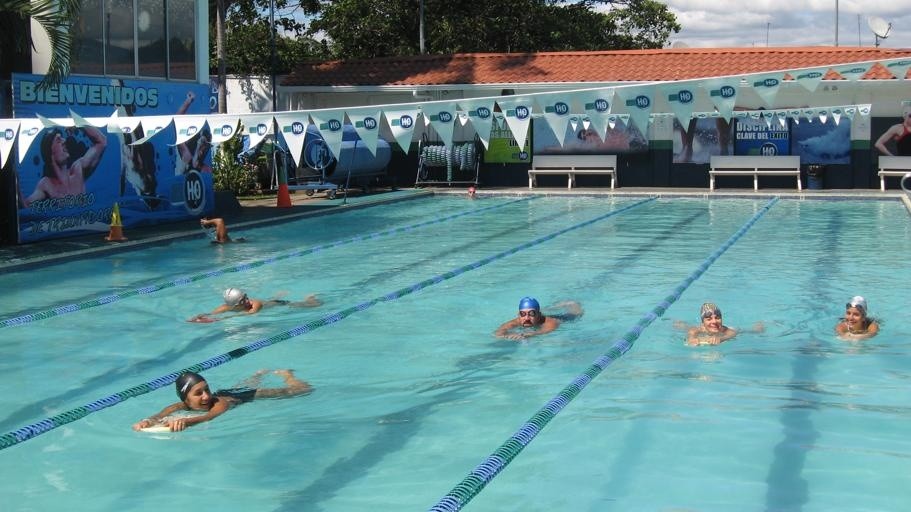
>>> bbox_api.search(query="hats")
[176,371,206,402]
[223,287,246,307]
[41,129,62,165]
[847,296,866,318]
[518,297,540,310]
[700,303,721,321]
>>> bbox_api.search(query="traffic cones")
[104,201,130,242]
[276,166,296,208]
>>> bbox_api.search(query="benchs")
[708,155,802,191]
[877,155,910,190]
[527,154,619,191]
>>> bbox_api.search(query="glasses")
[238,294,248,305]
[197,137,212,149]
[704,310,721,317]
[519,310,536,317]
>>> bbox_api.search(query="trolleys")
[270,149,344,199]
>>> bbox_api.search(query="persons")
[547,127,630,151]
[115,80,166,208]
[834,296,880,340]
[875,105,910,155]
[677,118,733,163]
[132,368,314,431]
[187,287,322,323]
[200,217,245,247]
[18,125,108,208]
[175,91,213,176]
[464,186,480,199]
[490,295,581,340]
[663,302,763,347]
